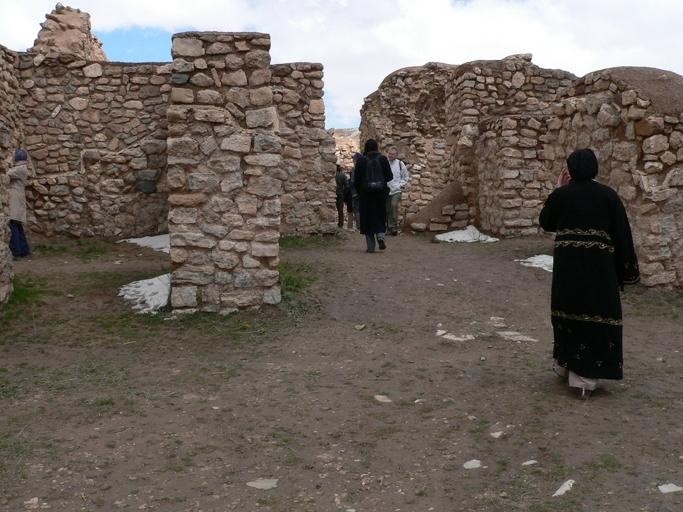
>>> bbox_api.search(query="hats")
[350,153,362,159]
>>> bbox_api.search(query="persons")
[353,138,393,253]
[348,151,364,234]
[538,146,642,401]
[6,149,32,261]
[333,158,348,228]
[384,145,410,236]
[344,170,357,230]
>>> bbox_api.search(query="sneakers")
[383,229,403,236]
[366,240,388,253]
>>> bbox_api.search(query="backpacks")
[363,154,385,190]
